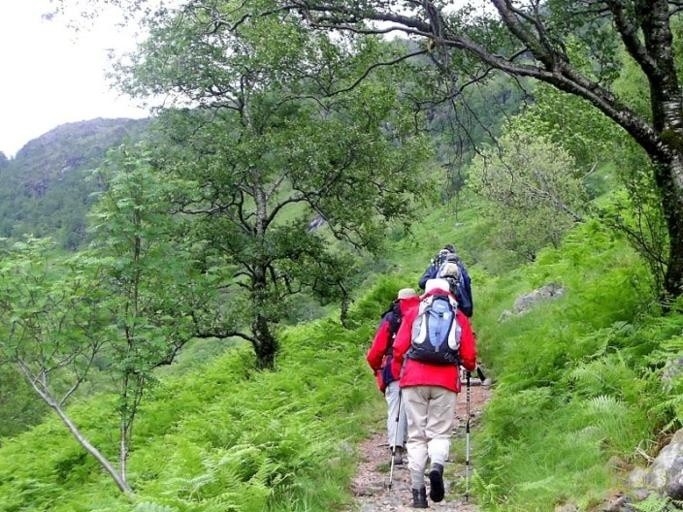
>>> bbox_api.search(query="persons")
[368,286,421,471]
[387,278,478,510]
[418,243,475,318]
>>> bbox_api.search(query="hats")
[439,244,457,260]
[397,287,417,301]
[424,278,451,294]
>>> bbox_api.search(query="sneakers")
[412,486,428,509]
[390,448,403,465]
[429,462,446,503]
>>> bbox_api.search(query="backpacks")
[440,263,459,287]
[410,291,463,365]
